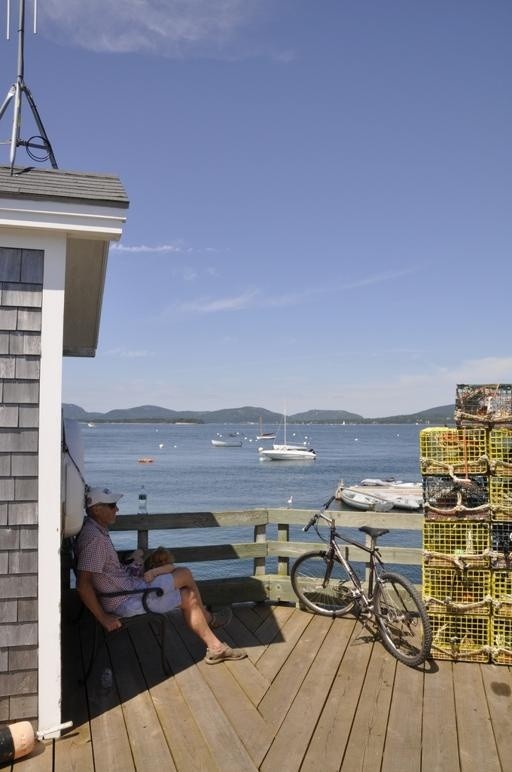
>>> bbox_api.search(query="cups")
[99,503,116,509]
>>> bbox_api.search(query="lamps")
[63,533,168,684]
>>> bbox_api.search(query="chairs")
[203,642,248,665]
[208,608,233,630]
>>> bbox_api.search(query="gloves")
[335,477,423,512]
[138,458,154,463]
[210,409,316,460]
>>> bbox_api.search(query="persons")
[75,487,247,664]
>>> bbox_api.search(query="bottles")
[87,487,123,507]
